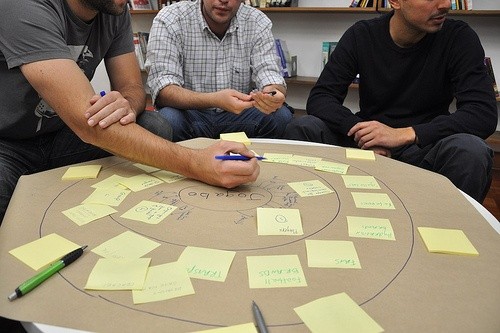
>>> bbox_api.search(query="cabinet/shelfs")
[130,0,500,112]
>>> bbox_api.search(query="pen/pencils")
[249,91,277,101]
[8,244,88,300]
[252,300,268,333]
[215,155,267,161]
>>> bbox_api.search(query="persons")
[0,0,261,219]
[284,0,498,205]
[142,0,293,139]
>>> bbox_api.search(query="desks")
[0,138,500,333]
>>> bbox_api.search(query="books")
[348,0,396,11]
[131,32,149,72]
[450,0,472,10]
[485,56,496,89]
[273,38,295,79]
[127,0,293,11]
[331,41,338,56]
[321,40,329,67]
